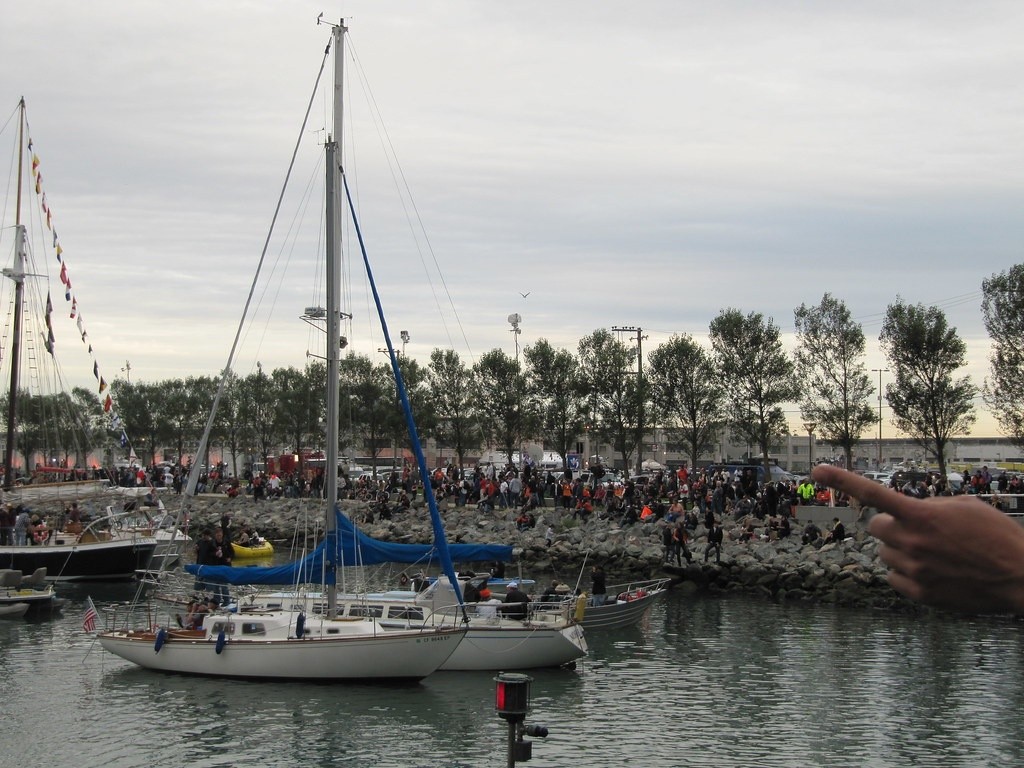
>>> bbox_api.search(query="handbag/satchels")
[681,551,693,559]
[463,480,470,490]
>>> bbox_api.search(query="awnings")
[479,450,563,465]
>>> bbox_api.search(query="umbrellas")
[633,457,667,471]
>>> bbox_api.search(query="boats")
[0,584,70,621]
[229,538,275,560]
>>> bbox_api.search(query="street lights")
[804,421,817,481]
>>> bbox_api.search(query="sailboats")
[95,11,673,688]
[1,95,194,582]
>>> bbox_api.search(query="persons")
[810,463,1024,616]
[491,559,505,579]
[185,526,235,631]
[0,457,1024,566]
[494,583,533,620]
[541,580,563,610]
[590,566,607,606]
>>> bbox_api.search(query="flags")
[83,596,98,632]
[399,572,410,587]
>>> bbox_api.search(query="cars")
[857,468,1024,499]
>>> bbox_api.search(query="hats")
[209,597,220,605]
[505,582,518,588]
[23,506,32,511]
[271,474,277,479]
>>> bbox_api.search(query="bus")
[706,464,793,490]
